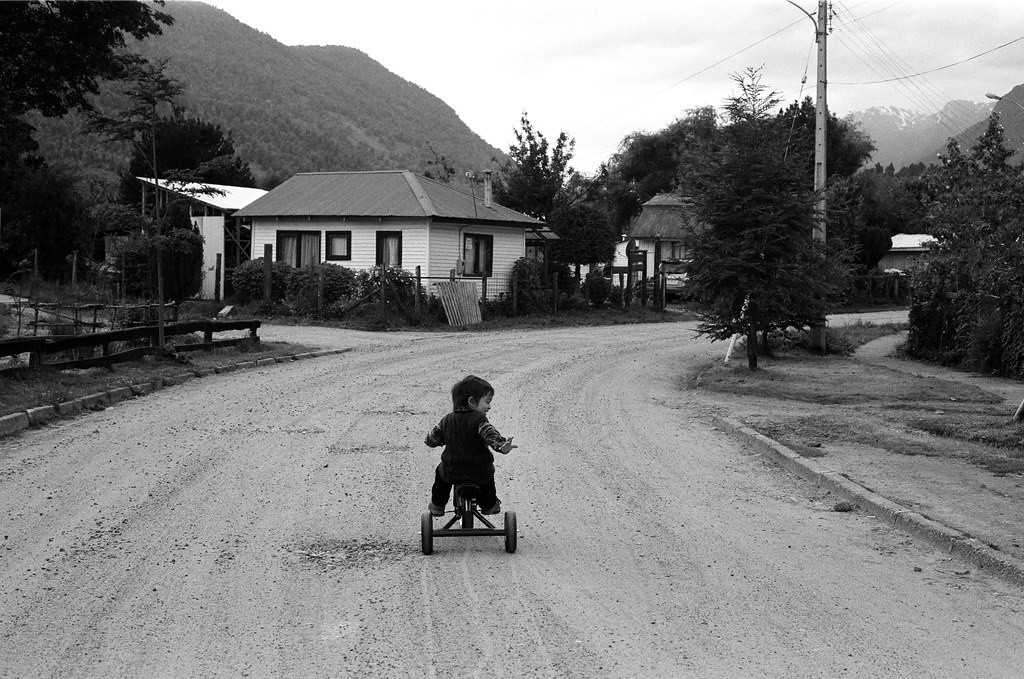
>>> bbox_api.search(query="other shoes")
[428,501,444,516]
[481,497,502,515]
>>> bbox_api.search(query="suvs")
[658,256,696,302]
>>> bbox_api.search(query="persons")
[425,375,517,516]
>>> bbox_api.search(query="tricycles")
[421,481,518,555]
